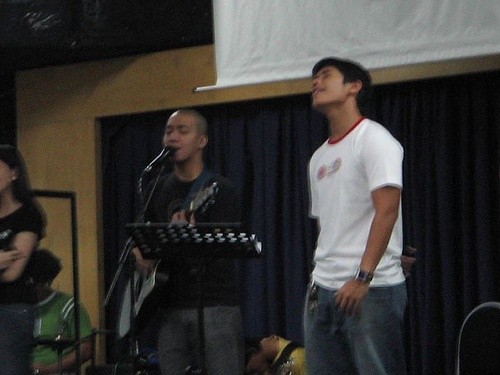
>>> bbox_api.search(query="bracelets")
[354,269,374,282]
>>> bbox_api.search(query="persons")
[0,144,47,375]
[130,109,246,375]
[26,250,93,375]
[245,335,306,375]
[304,57,408,375]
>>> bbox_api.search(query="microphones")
[145,143,178,175]
